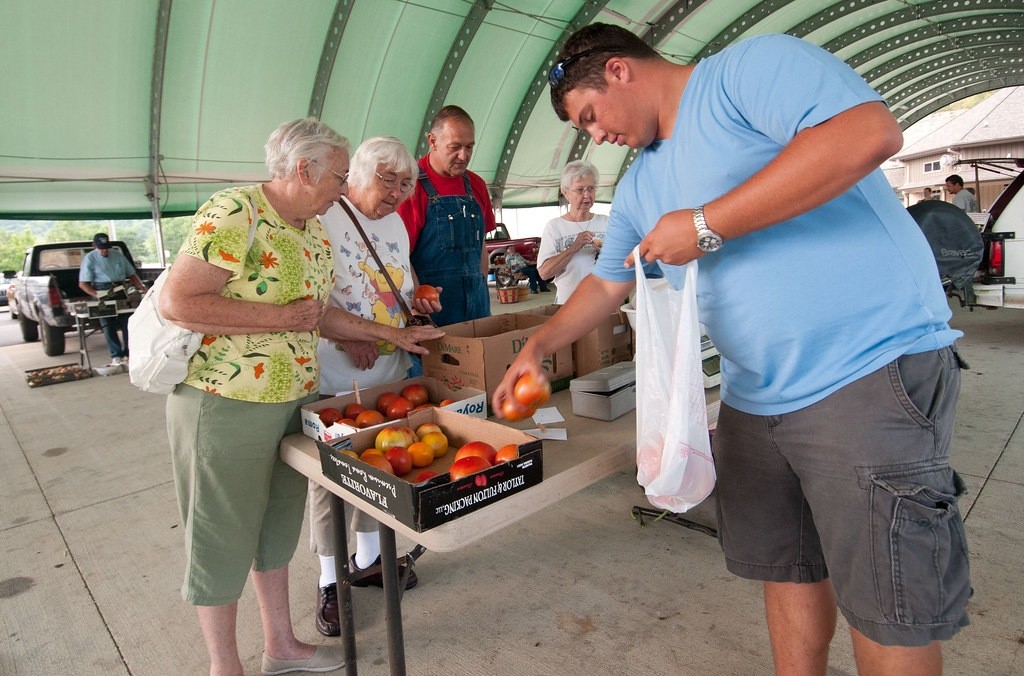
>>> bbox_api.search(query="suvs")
[484,223,541,287]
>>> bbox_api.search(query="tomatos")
[318,384,455,429]
[337,424,522,483]
[500,372,552,421]
[414,284,438,303]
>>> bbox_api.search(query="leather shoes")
[315,577,341,637]
[349,553,418,590]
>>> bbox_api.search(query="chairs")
[509,264,530,288]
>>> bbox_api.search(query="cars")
[962,160,1024,310]
[0,272,21,319]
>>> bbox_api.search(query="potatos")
[29,367,84,384]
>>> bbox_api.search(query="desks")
[75,308,136,379]
[282,386,723,676]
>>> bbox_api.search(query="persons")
[159,117,446,676]
[491,21,977,676]
[537,160,611,306]
[310,137,442,638]
[395,104,497,376]
[945,174,979,214]
[917,187,936,203]
[504,245,552,294]
[79,232,149,366]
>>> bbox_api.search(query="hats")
[94,233,112,249]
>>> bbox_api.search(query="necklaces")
[568,212,591,232]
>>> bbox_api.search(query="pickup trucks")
[15,241,165,357]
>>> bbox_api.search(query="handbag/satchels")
[127,188,258,395]
[633,244,717,514]
[407,314,438,359]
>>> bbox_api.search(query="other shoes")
[261,644,345,676]
[531,289,538,294]
[540,288,550,292]
[112,355,129,364]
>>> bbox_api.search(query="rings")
[321,306,326,313]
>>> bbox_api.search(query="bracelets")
[138,284,145,289]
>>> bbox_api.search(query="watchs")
[691,204,724,254]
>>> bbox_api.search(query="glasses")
[548,47,622,90]
[375,170,414,193]
[566,185,598,194]
[309,157,349,187]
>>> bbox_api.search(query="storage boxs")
[517,303,632,379]
[301,374,488,443]
[97,284,128,300]
[88,300,118,319]
[315,405,543,534]
[569,361,636,423]
[25,362,92,389]
[67,301,88,316]
[419,311,574,419]
